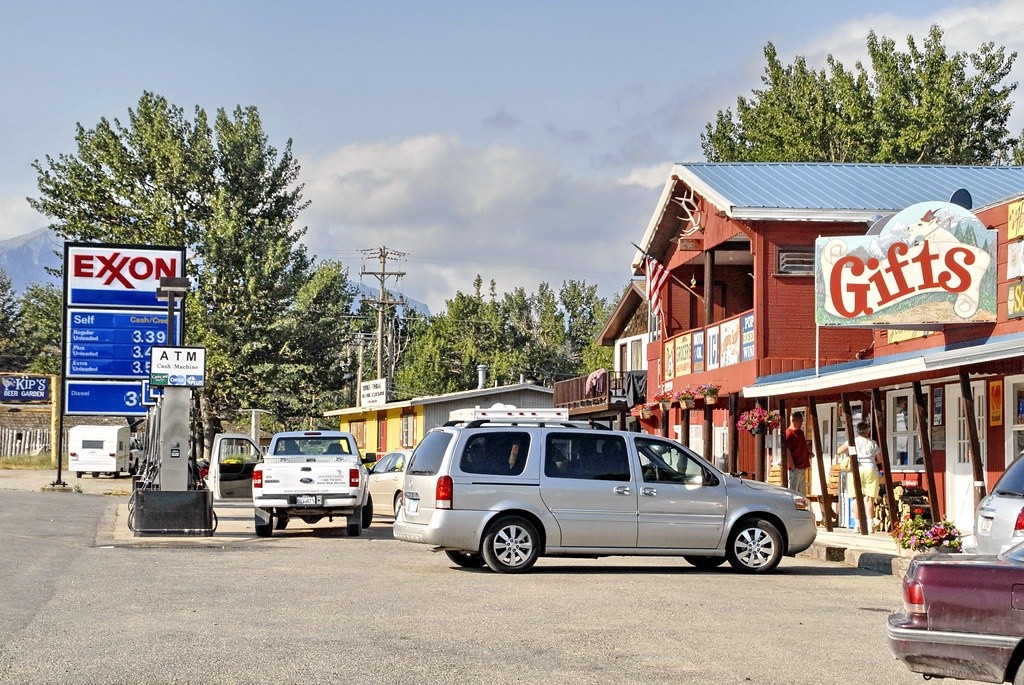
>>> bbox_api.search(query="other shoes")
[869,526,875,533]
[854,526,860,533]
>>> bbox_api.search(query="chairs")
[324,443,347,454]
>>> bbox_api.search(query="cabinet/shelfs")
[894,486,930,523]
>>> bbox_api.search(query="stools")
[221,472,238,481]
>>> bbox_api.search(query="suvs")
[392,407,817,574]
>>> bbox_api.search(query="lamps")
[690,259,696,288]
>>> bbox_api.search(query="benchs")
[767,465,842,526]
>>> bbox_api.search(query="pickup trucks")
[206,431,375,537]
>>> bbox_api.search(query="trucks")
[67,424,146,479]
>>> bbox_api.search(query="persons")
[836,422,883,534]
[784,411,814,499]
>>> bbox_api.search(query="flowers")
[673,386,697,401]
[695,384,724,396]
[890,509,963,551]
[735,407,781,432]
[635,403,654,415]
[654,390,674,403]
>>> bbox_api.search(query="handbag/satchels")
[840,456,852,472]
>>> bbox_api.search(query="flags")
[644,256,669,318]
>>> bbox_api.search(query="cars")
[886,541,1024,685]
[365,450,414,523]
[973,451,1024,555]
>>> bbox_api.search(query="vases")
[752,422,767,435]
[680,400,699,410]
[658,402,674,410]
[936,547,958,553]
[704,395,718,405]
[639,411,649,419]
